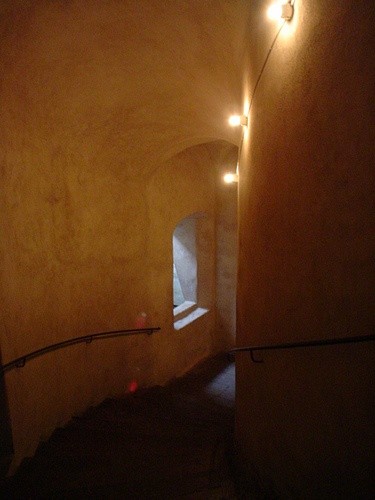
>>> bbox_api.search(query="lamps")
[228,114,248,126]
[267,1,293,21]
[223,172,238,184]
